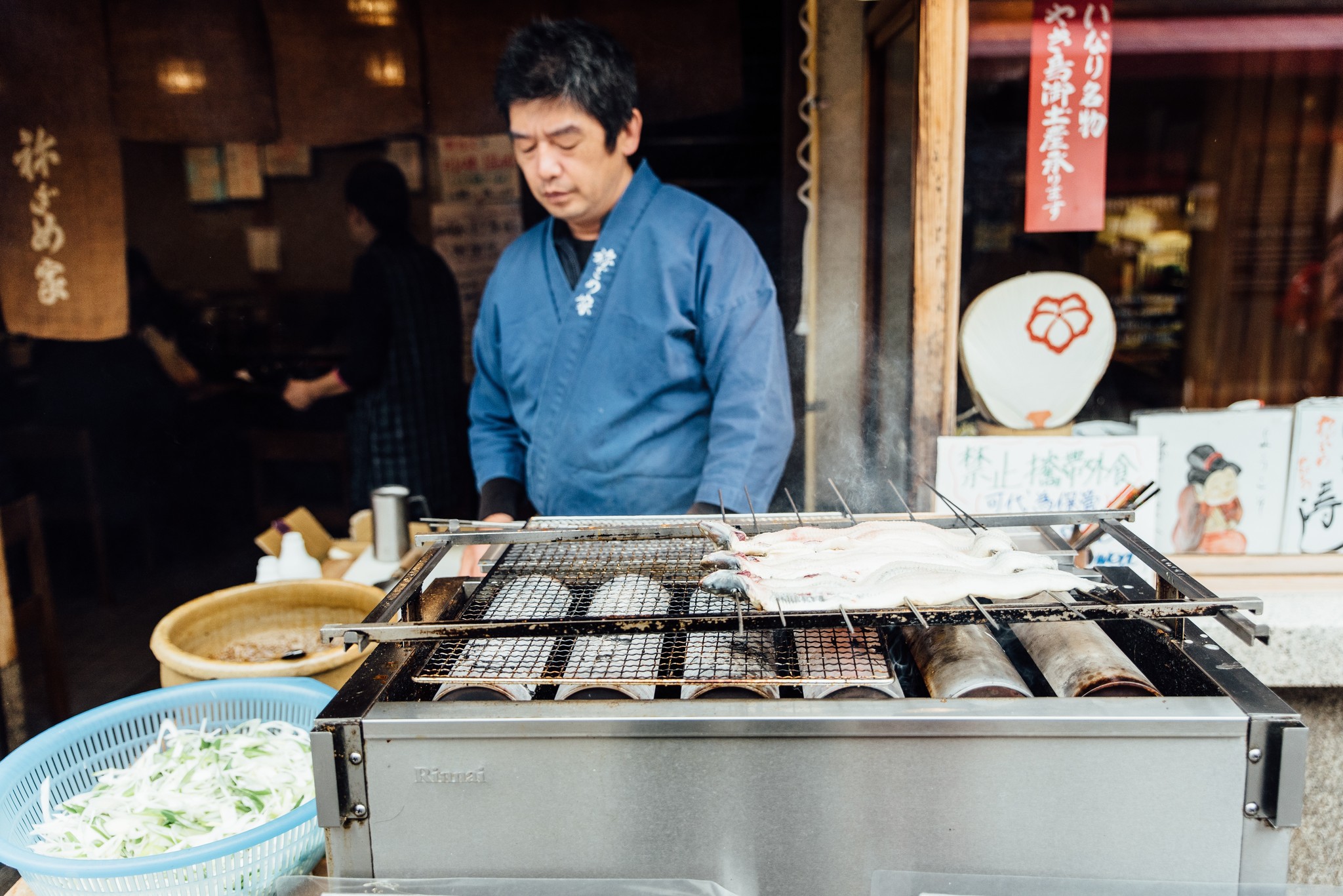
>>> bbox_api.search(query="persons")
[282,157,478,517]
[466,21,793,523]
[35,236,205,600]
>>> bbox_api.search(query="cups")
[368,482,411,561]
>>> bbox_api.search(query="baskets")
[0,678,335,896]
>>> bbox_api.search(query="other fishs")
[696,517,1120,612]
